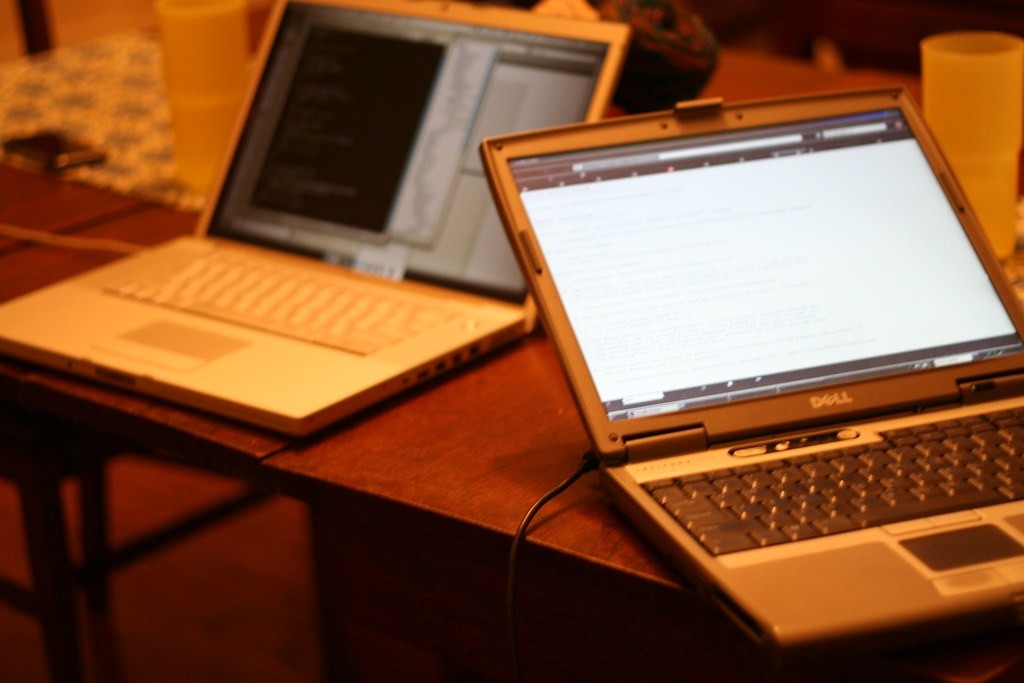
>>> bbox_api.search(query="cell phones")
[2,129,105,170]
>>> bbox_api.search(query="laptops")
[0,0,631,438]
[480,84,1024,650]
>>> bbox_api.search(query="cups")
[920,30,1024,258]
[153,0,252,186]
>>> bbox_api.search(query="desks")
[1,0,1024,683]
